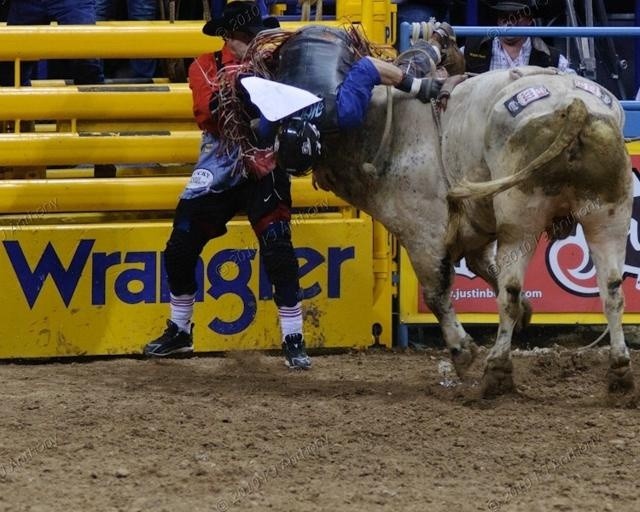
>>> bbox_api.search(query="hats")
[203,1,280,36]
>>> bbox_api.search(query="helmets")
[273,116,322,176]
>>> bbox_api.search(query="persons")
[141,2,312,371]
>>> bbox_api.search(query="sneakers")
[143,320,196,358]
[281,333,312,369]
[432,21,466,77]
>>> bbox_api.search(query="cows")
[257,16,636,402]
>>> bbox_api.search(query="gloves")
[395,76,442,104]
